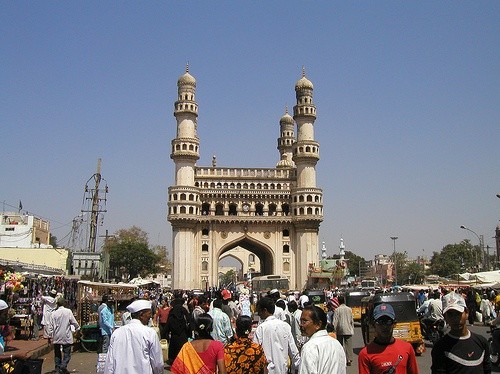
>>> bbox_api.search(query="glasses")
[374,319,394,325]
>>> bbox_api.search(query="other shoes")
[347,361,351,366]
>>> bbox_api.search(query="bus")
[361,280,375,295]
[252,275,290,301]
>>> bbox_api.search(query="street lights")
[460,226,481,272]
[390,236,399,285]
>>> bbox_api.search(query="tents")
[458,270,500,290]
[409,283,439,292]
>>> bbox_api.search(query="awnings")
[0,258,66,277]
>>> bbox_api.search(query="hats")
[442,292,467,314]
[126,300,152,312]
[370,304,396,320]
[270,289,278,294]
[0,299,8,310]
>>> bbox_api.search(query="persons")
[430,291,491,374]
[96,286,354,374]
[41,289,64,348]
[376,287,500,356]
[44,297,83,374]
[0,299,10,356]
[358,303,419,374]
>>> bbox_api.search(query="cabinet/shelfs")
[11,304,35,340]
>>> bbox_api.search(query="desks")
[81,325,121,350]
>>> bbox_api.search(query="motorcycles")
[417,311,444,345]
[302,290,325,312]
[486,322,500,367]
[361,292,425,356]
[343,291,370,323]
